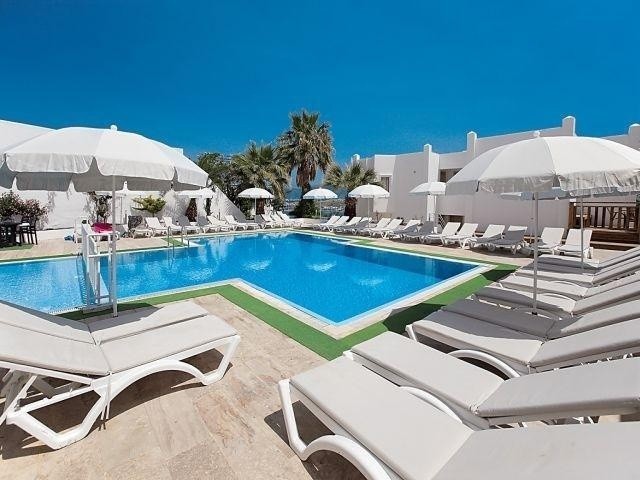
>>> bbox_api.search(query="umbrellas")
[178,247,218,284]
[409,180,446,226]
[500,183,639,274]
[302,186,338,220]
[349,247,386,288]
[94,180,173,224]
[0,124,209,317]
[348,182,390,228]
[299,242,336,272]
[175,188,215,225]
[445,132,640,315]
[237,184,274,223]
[239,238,273,272]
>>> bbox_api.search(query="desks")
[4,221,21,246]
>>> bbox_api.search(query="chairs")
[406,310,640,374]
[471,246,640,320]
[16,216,31,243]
[72,210,322,240]
[1,302,206,397]
[278,357,640,479]
[2,314,243,451]
[19,215,38,245]
[343,331,639,431]
[442,298,640,337]
[326,213,595,262]
